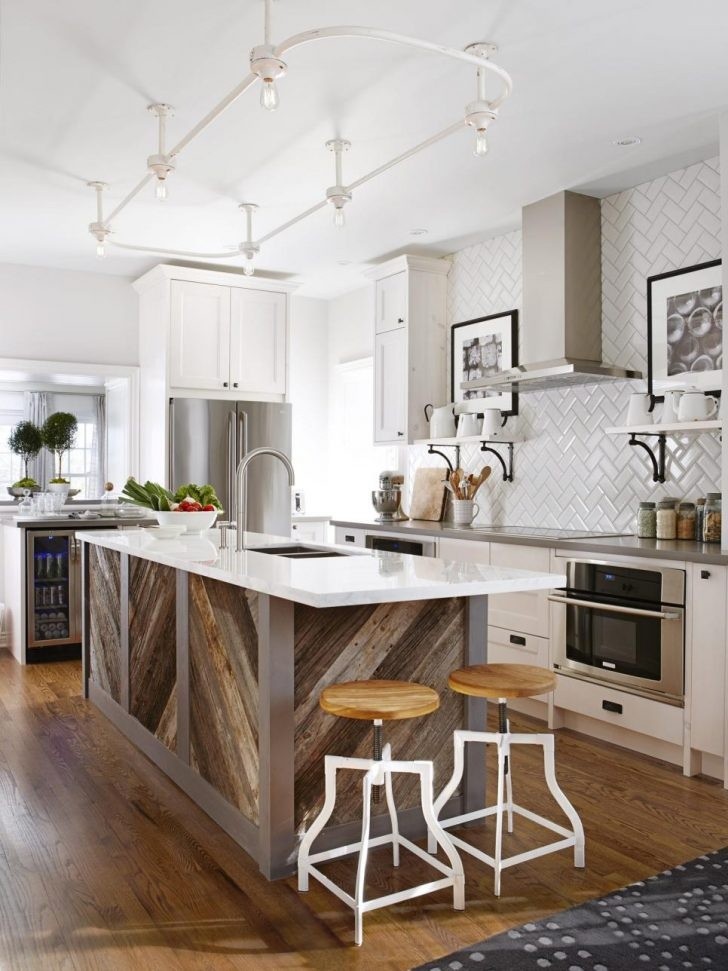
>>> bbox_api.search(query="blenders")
[371,472,409,522]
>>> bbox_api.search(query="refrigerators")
[169,397,292,538]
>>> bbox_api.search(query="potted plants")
[6,412,79,497]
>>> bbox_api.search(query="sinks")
[277,551,350,558]
[242,542,328,554]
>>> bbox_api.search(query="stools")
[298,680,465,940]
[428,664,585,900]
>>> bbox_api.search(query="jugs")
[456,412,480,437]
[482,409,507,435]
[424,402,457,438]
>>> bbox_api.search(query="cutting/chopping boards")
[409,467,451,521]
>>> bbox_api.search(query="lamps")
[147,103,178,201]
[464,43,498,160]
[87,181,111,258]
[237,204,260,275]
[250,0,288,112]
[325,140,352,230]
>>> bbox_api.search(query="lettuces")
[173,484,222,509]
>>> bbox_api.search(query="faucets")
[215,519,237,550]
[233,446,296,553]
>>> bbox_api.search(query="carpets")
[408,842,728,971]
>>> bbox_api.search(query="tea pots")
[671,386,719,422]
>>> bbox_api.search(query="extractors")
[460,190,643,390]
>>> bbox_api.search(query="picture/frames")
[646,258,722,402]
[451,310,519,417]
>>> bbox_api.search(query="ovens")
[548,557,687,708]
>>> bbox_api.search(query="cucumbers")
[150,494,171,511]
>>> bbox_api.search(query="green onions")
[119,479,174,507]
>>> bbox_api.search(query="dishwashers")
[366,535,436,557]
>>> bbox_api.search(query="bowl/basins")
[151,511,218,534]
[145,526,187,539]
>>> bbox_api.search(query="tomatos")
[173,501,214,511]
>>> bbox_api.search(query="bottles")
[677,502,695,539]
[637,502,656,537]
[695,499,706,542]
[17,489,32,517]
[655,502,677,539]
[100,482,118,516]
[702,492,721,542]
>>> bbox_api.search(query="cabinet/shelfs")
[131,264,288,403]
[439,538,728,790]
[373,254,453,444]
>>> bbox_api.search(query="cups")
[32,491,68,514]
[627,393,655,426]
[663,390,684,423]
[452,500,480,526]
